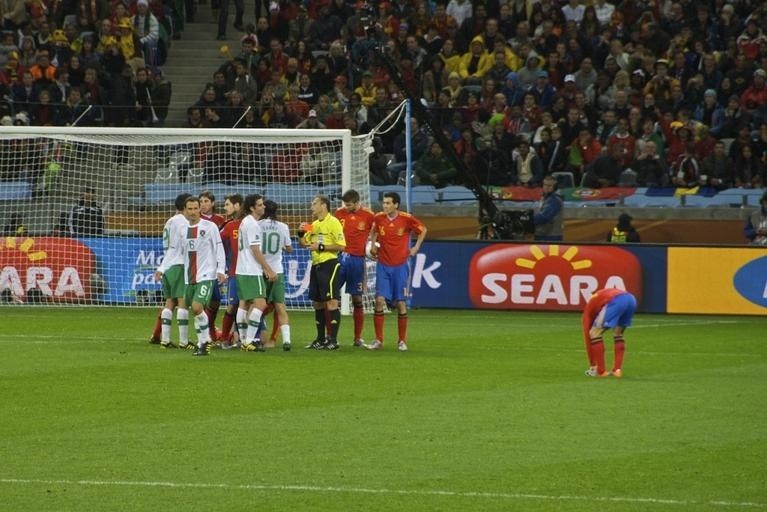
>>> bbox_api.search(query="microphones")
[538,192,544,196]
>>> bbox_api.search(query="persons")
[53,213,69,234]
[522,269,637,376]
[534,175,566,239]
[72,188,104,233]
[0,0,767,199]
[3,213,28,236]
[145,190,428,354]
[738,192,766,247]
[602,213,641,243]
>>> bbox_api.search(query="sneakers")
[215,332,275,352]
[398,340,408,351]
[586,369,622,377]
[148,336,213,355]
[355,339,384,350]
[305,337,340,351]
[283,343,291,350]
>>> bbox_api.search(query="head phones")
[541,176,558,190]
